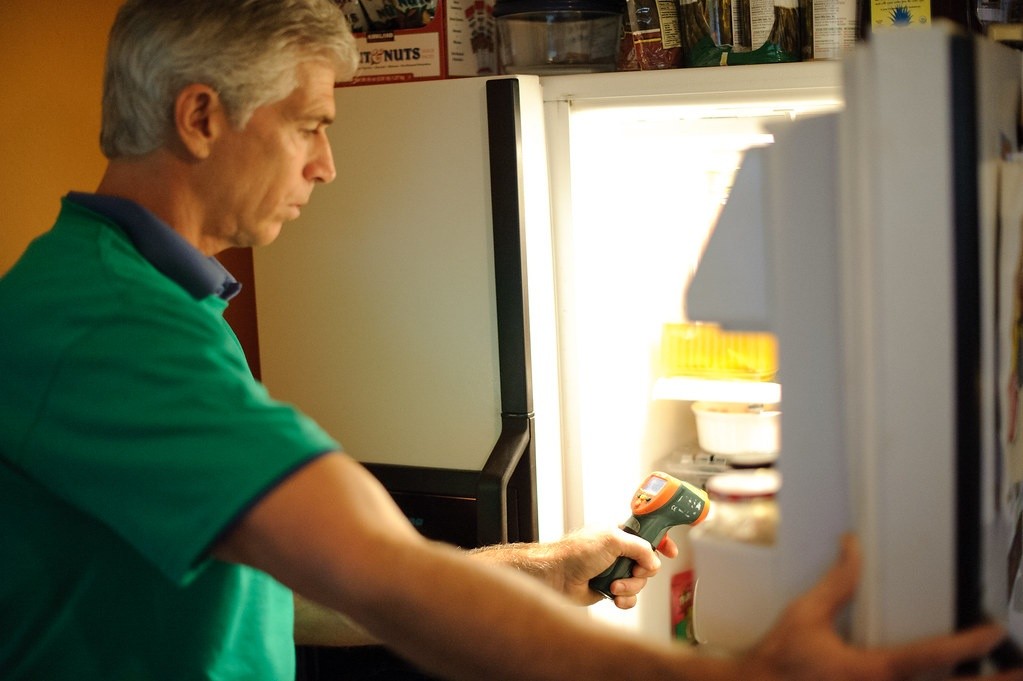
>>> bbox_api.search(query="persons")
[0,0,1006,681]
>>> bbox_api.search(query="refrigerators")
[254,19,1023,681]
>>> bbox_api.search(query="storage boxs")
[331,0,500,90]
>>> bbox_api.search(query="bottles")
[689,468,784,646]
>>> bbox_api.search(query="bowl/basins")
[691,403,780,465]
[490,1,625,74]
